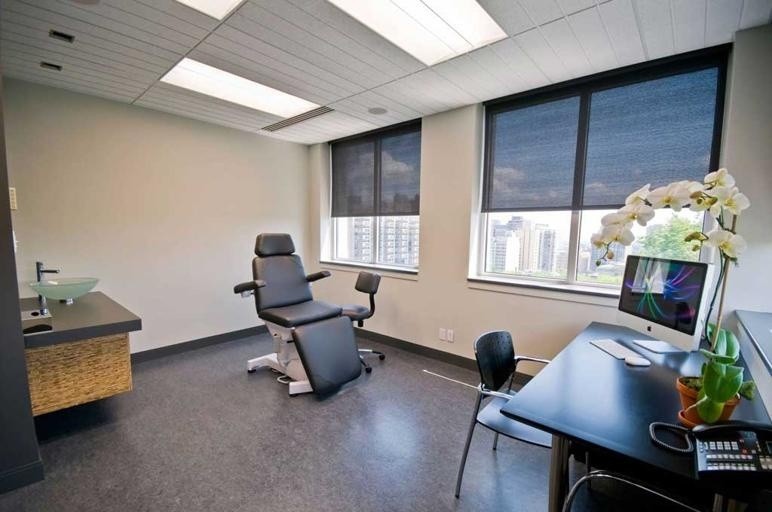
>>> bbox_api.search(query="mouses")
[625,355,652,367]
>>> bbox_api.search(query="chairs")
[340,270,386,373]
[455,330,591,499]
[234,232,361,399]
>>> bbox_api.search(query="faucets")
[36,261,60,303]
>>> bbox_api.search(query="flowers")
[590,169,752,421]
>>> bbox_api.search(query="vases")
[677,374,742,427]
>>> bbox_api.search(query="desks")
[500,321,771,512]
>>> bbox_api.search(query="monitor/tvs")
[616,253,716,355]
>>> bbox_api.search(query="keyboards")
[589,338,640,359]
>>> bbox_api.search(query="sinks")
[29,277,97,300]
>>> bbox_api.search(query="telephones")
[691,420,770,479]
